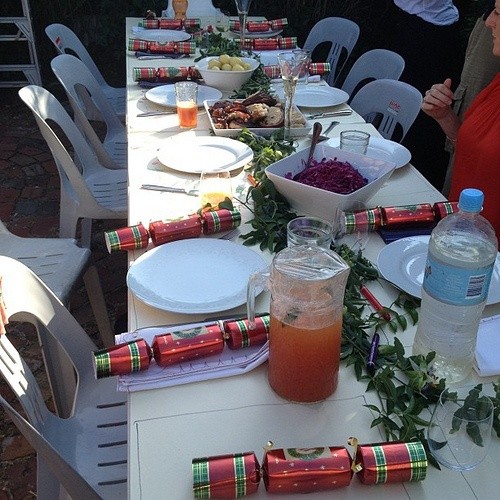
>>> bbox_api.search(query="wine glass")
[235,0,257,58]
[275,51,307,148]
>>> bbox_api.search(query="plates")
[377,234,500,306]
[327,135,411,171]
[126,237,271,313]
[202,97,312,139]
[155,135,253,174]
[146,84,223,107]
[133,28,190,42]
[234,26,350,108]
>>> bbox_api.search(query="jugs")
[247,217,349,403]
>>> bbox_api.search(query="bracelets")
[175,11,185,15]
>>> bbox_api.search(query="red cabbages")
[285,157,368,195]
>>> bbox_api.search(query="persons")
[101,0,187,55]
[212,0,500,254]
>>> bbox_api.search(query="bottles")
[410,188,497,382]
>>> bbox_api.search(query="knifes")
[137,106,203,118]
[306,108,353,120]
[142,184,198,196]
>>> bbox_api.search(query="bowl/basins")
[264,144,397,223]
[192,55,259,91]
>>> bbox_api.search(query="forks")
[138,53,186,60]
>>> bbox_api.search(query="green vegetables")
[228,63,272,99]
[230,129,306,252]
[331,237,500,471]
[193,31,263,62]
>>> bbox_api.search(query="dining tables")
[126,17,366,125]
[125,124,500,500]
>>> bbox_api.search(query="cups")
[215,8,230,32]
[175,81,199,128]
[286,217,333,249]
[427,384,494,469]
[292,49,311,82]
[172,0,188,20]
[339,130,370,156]
[198,167,232,209]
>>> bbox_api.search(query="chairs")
[0,15,500,500]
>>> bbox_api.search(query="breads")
[209,55,252,70]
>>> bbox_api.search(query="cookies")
[208,87,305,129]
